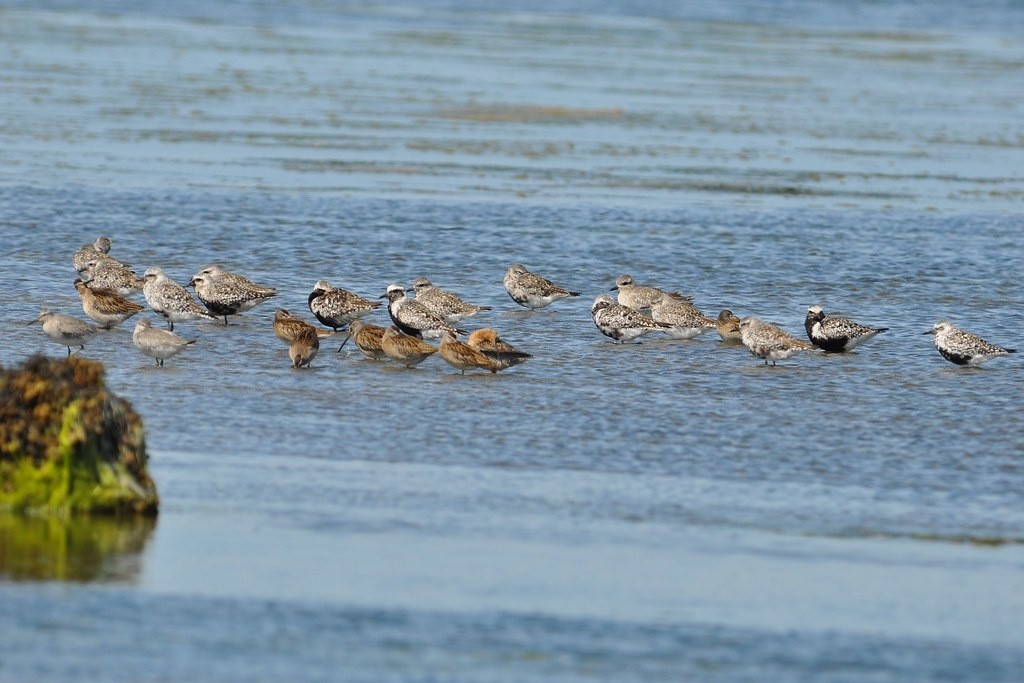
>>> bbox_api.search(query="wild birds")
[591,275,742,345]
[27,310,103,356]
[72,237,535,376]
[731,315,826,366]
[804,305,890,355]
[503,263,582,311]
[921,320,1018,367]
